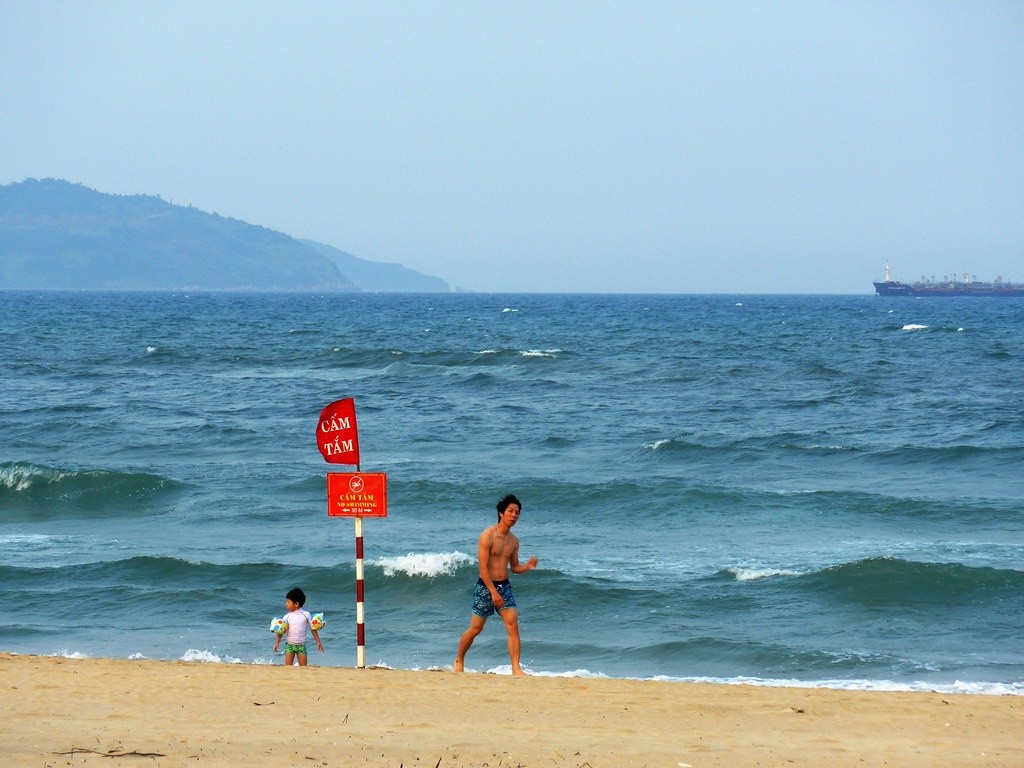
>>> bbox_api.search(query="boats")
[871,259,1024,298]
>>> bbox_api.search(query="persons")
[453,494,538,676]
[274,588,325,667]
